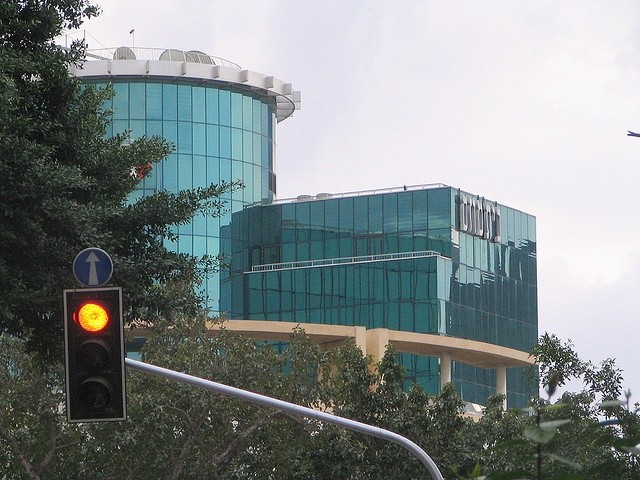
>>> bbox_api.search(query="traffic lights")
[65,288,126,422]
